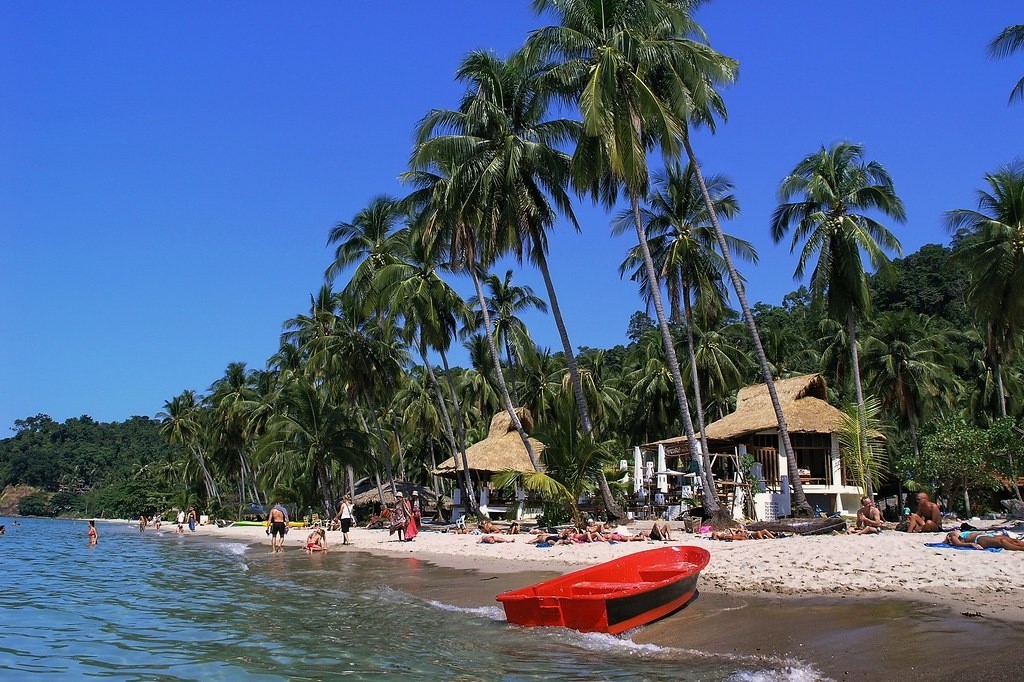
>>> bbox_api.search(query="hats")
[588,519,593,523]
[412,491,419,496]
[395,492,403,498]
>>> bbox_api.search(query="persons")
[449,520,521,544]
[875,504,900,522]
[711,528,794,541]
[334,495,357,545]
[12,521,20,526]
[302,528,328,554]
[139,515,145,533]
[176,506,198,532]
[390,491,421,543]
[523,517,679,547]
[303,513,356,531]
[833,496,881,537]
[200,513,230,528]
[266,502,290,554]
[156,514,162,532]
[944,530,1024,551]
[907,492,942,533]
[0,525,5,535]
[123,514,156,527]
[175,525,184,535]
[87,520,98,545]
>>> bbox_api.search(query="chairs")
[456,515,466,529]
[1000,499,1024,524]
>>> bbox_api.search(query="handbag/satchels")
[350,514,355,523]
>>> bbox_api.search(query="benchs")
[638,561,698,581]
[421,517,449,532]
[573,581,660,595]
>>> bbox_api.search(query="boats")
[494,545,711,633]
